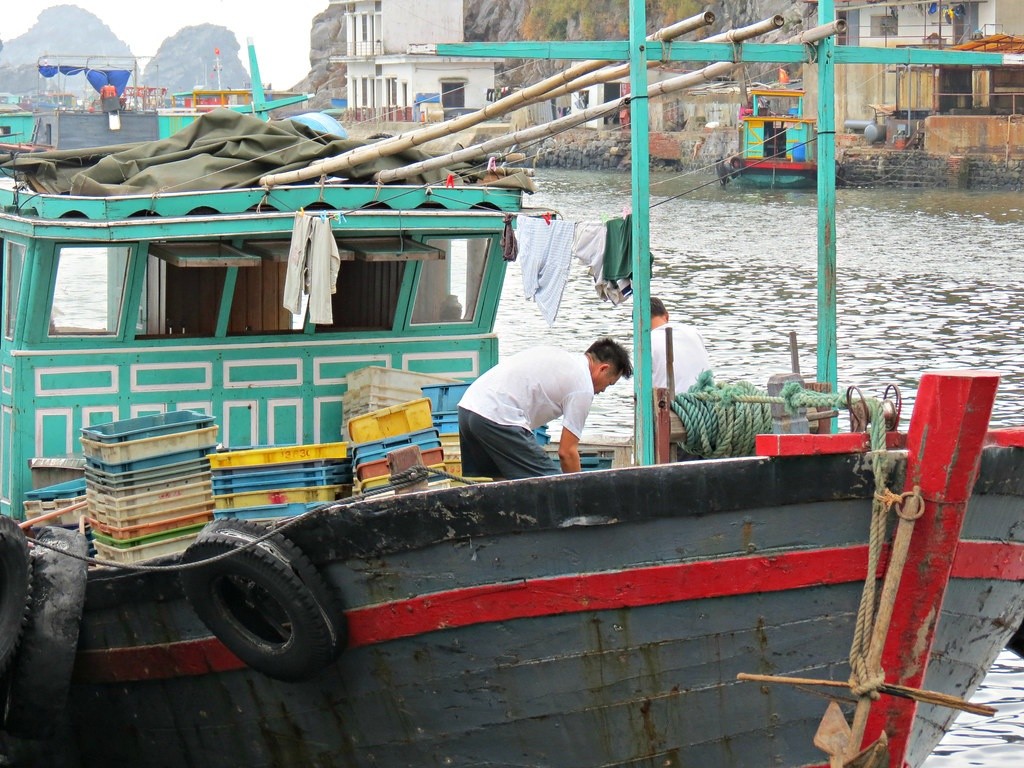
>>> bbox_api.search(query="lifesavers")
[178,516,344,684]
[730,156,743,172]
[716,160,729,186]
[0,512,90,744]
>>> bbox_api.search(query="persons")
[632,298,709,396]
[457,338,633,480]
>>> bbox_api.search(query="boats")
[0,0,1024,768]
[734,88,817,191]
[0,32,317,150]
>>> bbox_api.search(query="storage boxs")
[25,365,632,572]
